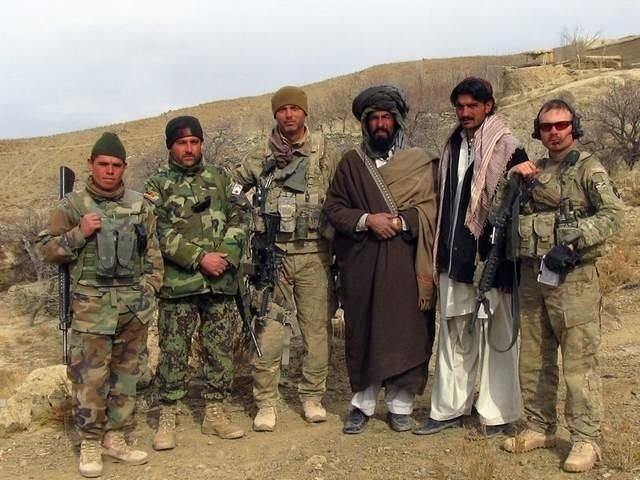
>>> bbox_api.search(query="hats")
[91,132,126,161]
[352,85,409,124]
[272,86,307,119]
[165,115,204,150]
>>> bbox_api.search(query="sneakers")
[302,400,328,423]
[252,406,277,432]
[502,426,558,453]
[562,437,599,472]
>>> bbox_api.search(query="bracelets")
[393,217,398,227]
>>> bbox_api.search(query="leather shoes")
[387,411,413,433]
[411,417,465,436]
[342,407,368,434]
[464,422,513,442]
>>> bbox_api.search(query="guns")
[469,173,522,334]
[236,273,264,358]
[60,166,75,365]
[246,191,279,341]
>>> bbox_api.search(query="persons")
[231,86,340,432]
[411,78,529,439]
[487,99,626,473]
[37,133,165,477]
[325,85,440,434]
[143,117,253,451]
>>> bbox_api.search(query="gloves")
[544,243,574,273]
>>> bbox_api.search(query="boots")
[152,404,178,450]
[102,430,148,466]
[79,439,104,478]
[200,398,245,439]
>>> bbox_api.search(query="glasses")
[539,121,571,133]
